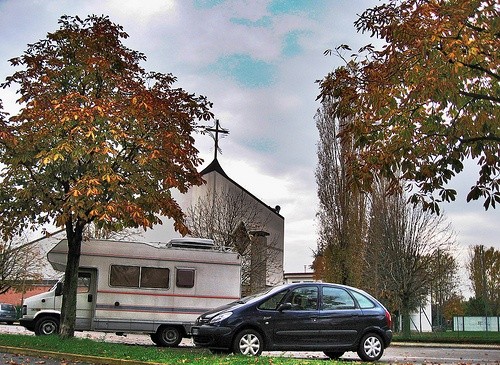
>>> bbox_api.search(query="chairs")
[292,294,303,309]
[304,298,316,310]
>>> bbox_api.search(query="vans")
[0,303,17,325]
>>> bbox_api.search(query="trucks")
[16,239,243,346]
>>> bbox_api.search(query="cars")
[191,281,393,362]
